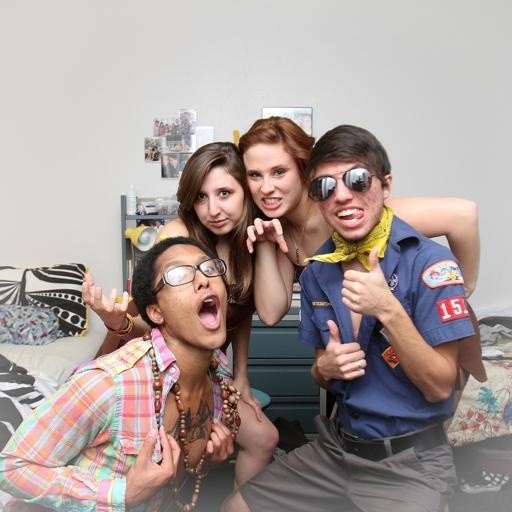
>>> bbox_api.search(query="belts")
[337,426,448,461]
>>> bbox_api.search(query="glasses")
[305,167,377,202]
[154,258,227,297]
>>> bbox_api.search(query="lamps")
[124,225,158,302]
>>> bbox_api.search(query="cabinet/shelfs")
[120,195,329,462]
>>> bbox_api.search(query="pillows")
[0,263,90,337]
[0,303,64,345]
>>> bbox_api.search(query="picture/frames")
[262,107,313,140]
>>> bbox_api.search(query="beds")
[443,315,512,512]
[1,313,117,512]
